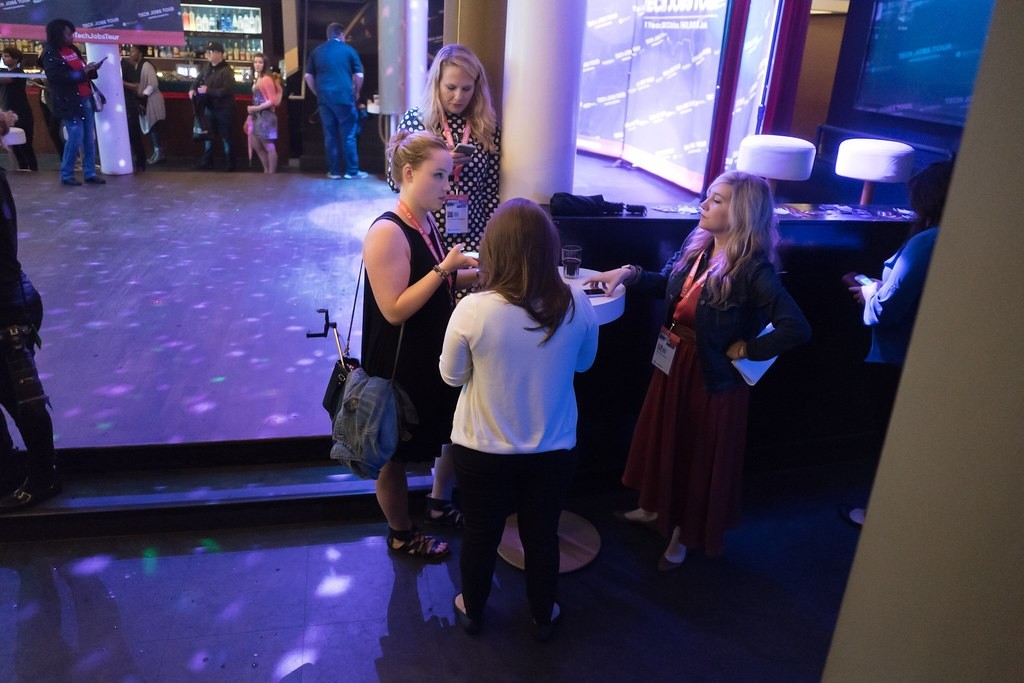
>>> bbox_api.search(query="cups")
[562,245,582,279]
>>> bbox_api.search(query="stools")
[736,134,817,204]
[835,137,915,204]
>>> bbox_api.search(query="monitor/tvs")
[851,1,993,132]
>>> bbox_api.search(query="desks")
[497,265,627,575]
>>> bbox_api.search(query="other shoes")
[0,465,63,512]
[658,542,688,574]
[84,176,107,184]
[327,172,341,179]
[344,170,368,179]
[849,507,865,525]
[61,177,82,185]
[613,510,658,530]
[190,158,214,170]
[225,162,235,170]
[453,584,482,636]
[530,590,561,643]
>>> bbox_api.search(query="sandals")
[386,522,449,559]
[424,493,468,530]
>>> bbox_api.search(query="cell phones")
[584,289,607,298]
[854,273,874,288]
[97,56,109,67]
[453,143,476,158]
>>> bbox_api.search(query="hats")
[204,42,224,53]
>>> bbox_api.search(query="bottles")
[0,38,40,53]
[121,39,263,61]
[182,7,261,33]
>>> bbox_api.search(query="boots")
[146,146,167,165]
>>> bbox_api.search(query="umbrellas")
[247,113,254,166]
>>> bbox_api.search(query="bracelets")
[433,263,449,281]
[621,264,637,282]
[738,342,744,360]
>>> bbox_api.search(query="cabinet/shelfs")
[120,0,276,82]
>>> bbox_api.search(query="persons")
[438,196,600,642]
[188,41,237,172]
[386,43,501,312]
[36,18,109,187]
[305,22,370,179]
[360,128,470,561]
[0,46,40,172]
[848,160,955,525]
[581,171,814,572]
[246,53,278,174]
[118,44,167,173]
[0,167,63,513]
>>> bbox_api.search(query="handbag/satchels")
[190,93,213,142]
[243,114,254,135]
[323,357,363,419]
[92,91,103,112]
